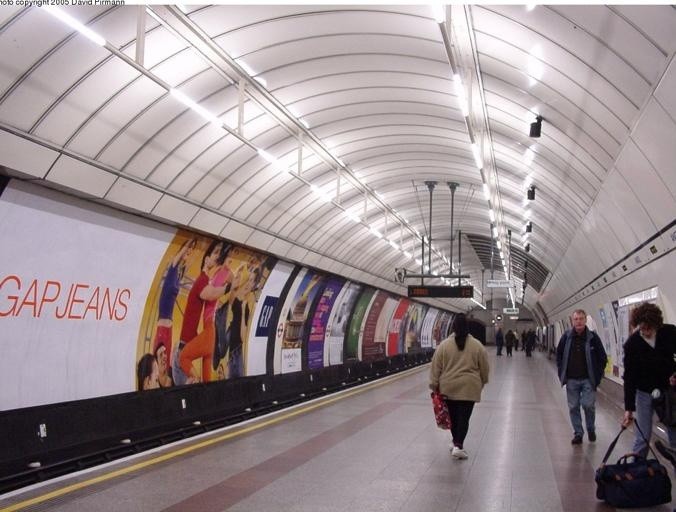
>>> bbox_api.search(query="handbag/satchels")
[431,391,452,429]
[595,453,672,509]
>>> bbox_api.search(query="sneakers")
[450,443,468,459]
[588,432,596,441]
[572,436,582,443]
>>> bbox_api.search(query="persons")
[429,311,490,461]
[619,301,676,469]
[136,233,263,392]
[554,308,610,445]
[495,326,541,358]
